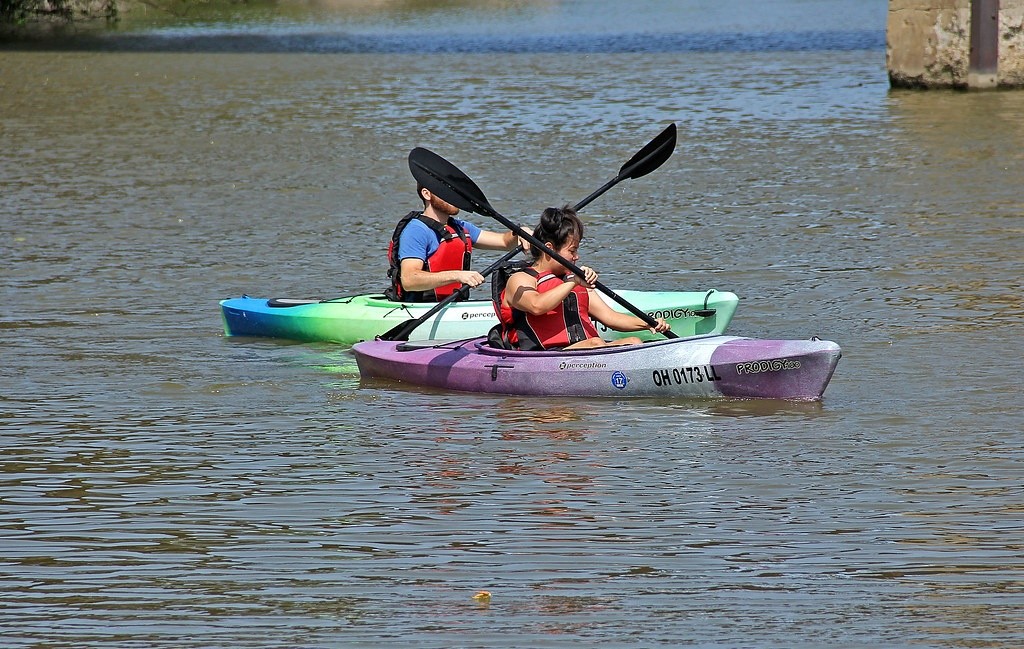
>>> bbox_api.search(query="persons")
[384,183,534,303]
[491,207,671,351]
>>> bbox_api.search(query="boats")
[217,287,742,350]
[352,332,841,405]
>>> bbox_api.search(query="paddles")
[378,122,679,342]
[408,147,682,338]
